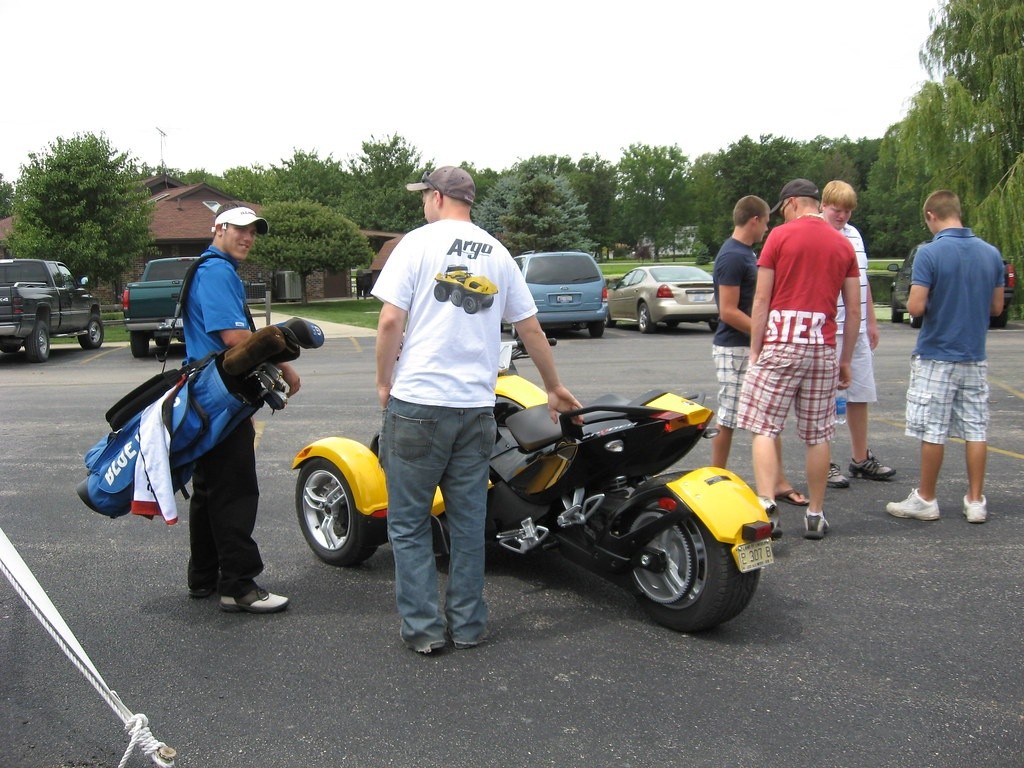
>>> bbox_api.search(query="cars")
[604,264,721,335]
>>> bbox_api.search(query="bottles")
[832,381,847,423]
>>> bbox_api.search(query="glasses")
[421,171,442,199]
[780,195,796,218]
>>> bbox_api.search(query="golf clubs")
[238,362,291,410]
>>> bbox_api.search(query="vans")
[501,248,609,339]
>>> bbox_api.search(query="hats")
[210,207,269,234]
[769,179,819,215]
[406,166,476,204]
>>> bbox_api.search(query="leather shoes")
[219,587,289,613]
[187,580,219,596]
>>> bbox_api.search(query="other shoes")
[759,498,782,537]
[803,508,831,539]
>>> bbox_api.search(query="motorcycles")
[292,335,783,635]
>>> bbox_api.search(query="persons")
[713,196,811,505]
[887,190,1004,523]
[370,166,584,652]
[738,178,861,539]
[820,180,896,488]
[181,203,301,614]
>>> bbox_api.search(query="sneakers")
[825,462,850,488]
[847,449,896,480]
[963,493,988,524]
[885,489,940,521]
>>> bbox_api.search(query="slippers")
[774,487,810,505]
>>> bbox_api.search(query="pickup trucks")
[886,239,1017,330]
[0,258,105,364]
[123,256,203,358]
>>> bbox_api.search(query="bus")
[640,225,703,259]
